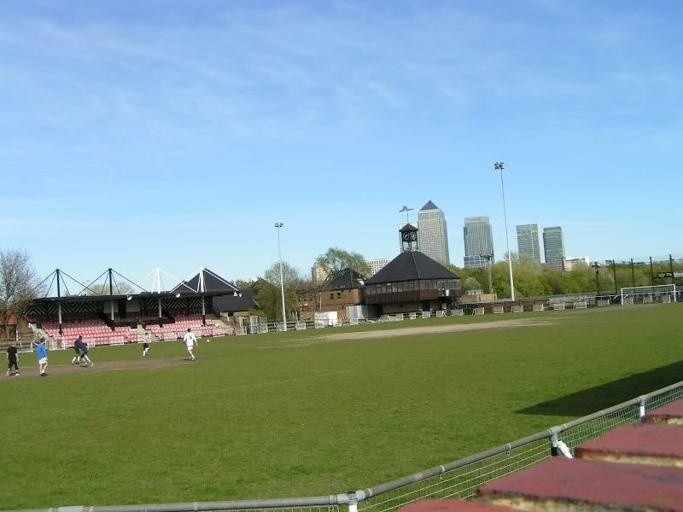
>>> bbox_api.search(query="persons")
[37,337,49,376]
[6,342,21,376]
[71,336,94,368]
[142,332,150,357]
[183,328,198,361]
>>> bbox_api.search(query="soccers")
[207,340,209,343]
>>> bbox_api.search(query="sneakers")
[40,371,48,376]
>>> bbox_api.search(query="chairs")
[25,308,225,348]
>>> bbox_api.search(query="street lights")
[271,219,289,333]
[493,159,517,304]
